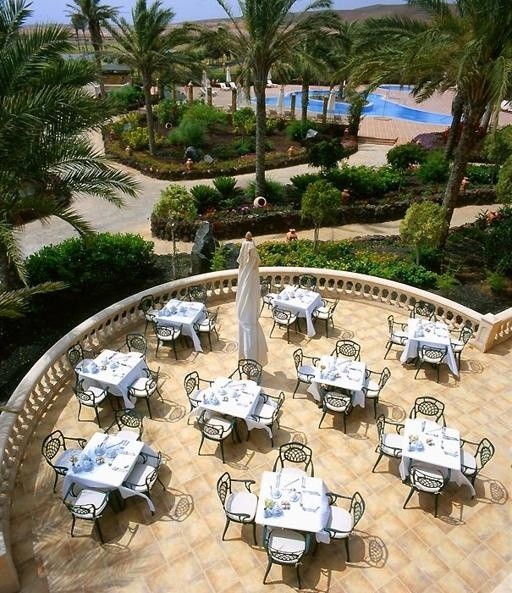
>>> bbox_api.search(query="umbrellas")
[236,231,269,372]
[226,65,231,82]
[201,70,209,91]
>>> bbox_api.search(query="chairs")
[41,408,165,546]
[182,360,285,465]
[140,286,220,362]
[67,332,164,419]
[257,271,340,346]
[376,397,495,521]
[385,300,474,384]
[217,442,365,591]
[293,339,392,434]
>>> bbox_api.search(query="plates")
[79,447,117,472]
[91,355,120,373]
[410,317,448,339]
[266,473,318,518]
[412,421,462,458]
[278,286,316,307]
[211,381,253,409]
[160,302,196,320]
[322,356,359,383]
